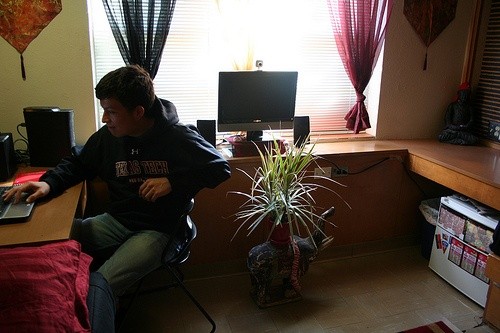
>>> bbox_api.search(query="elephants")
[246,206,336,309]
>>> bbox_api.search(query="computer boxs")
[23,106,75,167]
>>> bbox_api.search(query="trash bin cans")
[420,199,439,260]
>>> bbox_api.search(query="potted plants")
[216,124,358,252]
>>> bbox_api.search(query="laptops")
[0,186,36,222]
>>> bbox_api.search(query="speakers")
[0,132,17,181]
[197,120,216,147]
[293,116,310,147]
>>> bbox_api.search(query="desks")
[0,164,87,248]
[128,138,500,333]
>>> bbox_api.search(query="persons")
[3,66,232,302]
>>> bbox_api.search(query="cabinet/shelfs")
[333,167,349,176]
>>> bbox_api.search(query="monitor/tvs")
[217,71,299,141]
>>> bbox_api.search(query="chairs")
[116,189,218,333]
